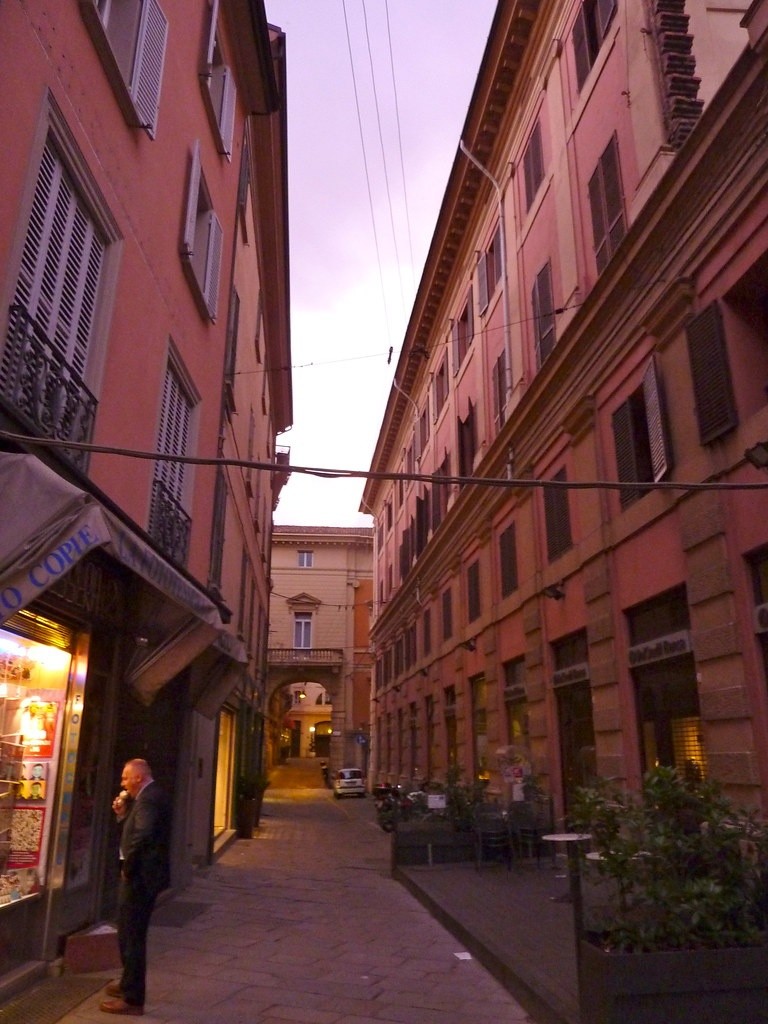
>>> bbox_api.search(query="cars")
[332,767,366,797]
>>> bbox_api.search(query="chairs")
[471,801,556,873]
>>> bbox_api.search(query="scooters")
[373,785,417,832]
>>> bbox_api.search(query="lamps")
[544,587,563,600]
[459,641,475,652]
[744,442,768,469]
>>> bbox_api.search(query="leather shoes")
[106,984,127,998]
[100,998,144,1016]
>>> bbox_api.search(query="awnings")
[0,451,218,702]
[129,626,249,721]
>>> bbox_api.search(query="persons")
[20,764,26,780]
[28,782,42,799]
[30,764,44,780]
[99,758,169,1016]
[16,782,25,800]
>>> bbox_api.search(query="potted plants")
[577,762,768,1024]
[237,763,269,839]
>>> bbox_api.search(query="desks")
[542,834,591,921]
[583,850,651,910]
